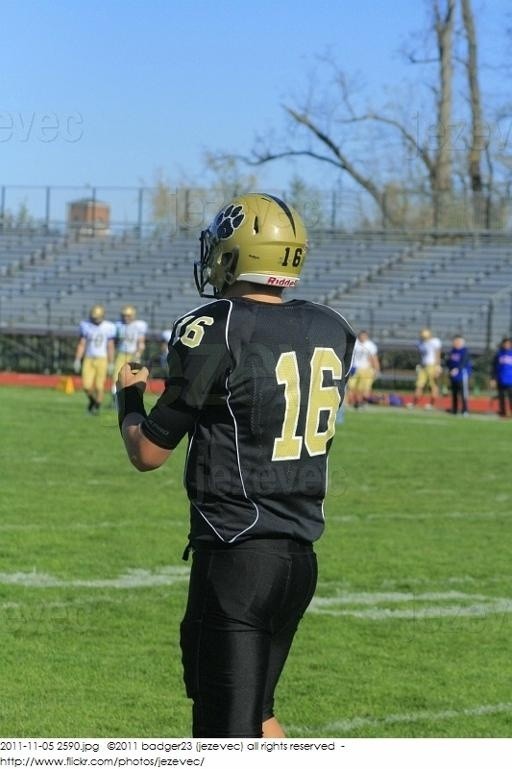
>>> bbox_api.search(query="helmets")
[193,192,309,301]
[88,304,106,324]
[119,304,137,324]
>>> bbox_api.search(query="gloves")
[73,357,82,375]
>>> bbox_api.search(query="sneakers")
[87,396,101,415]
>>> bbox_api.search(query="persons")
[113,303,148,396]
[409,326,442,411]
[159,328,173,380]
[492,339,511,418]
[112,190,356,737]
[346,327,381,409]
[346,334,360,407]
[440,335,472,418]
[74,304,120,416]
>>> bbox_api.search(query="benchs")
[0,224,512,369]
